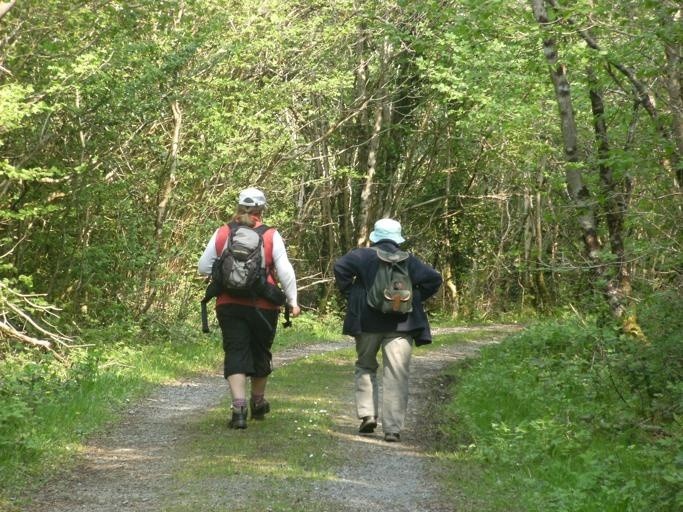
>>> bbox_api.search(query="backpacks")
[213,222,270,299]
[367,247,414,314]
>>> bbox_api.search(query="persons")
[197,187,302,429]
[333,216,444,441]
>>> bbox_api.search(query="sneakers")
[385,432,401,442]
[227,406,248,429]
[359,418,376,434]
[251,399,270,419]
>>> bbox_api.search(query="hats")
[239,188,268,212]
[369,219,405,245]
